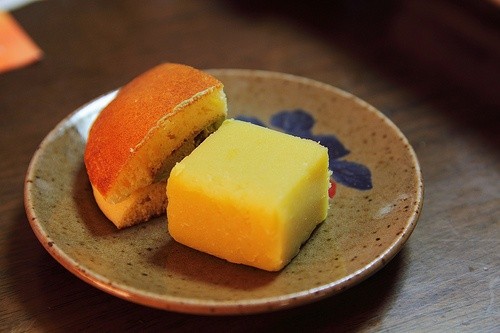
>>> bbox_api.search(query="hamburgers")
[84,62,228,229]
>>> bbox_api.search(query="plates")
[22,68,427,318]
